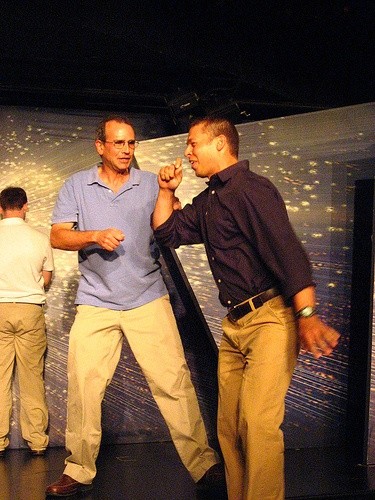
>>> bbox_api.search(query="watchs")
[295,305,320,319]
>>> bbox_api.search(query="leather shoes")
[195,463,227,487]
[45,474,95,496]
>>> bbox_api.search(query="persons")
[152,117,341,500]
[0,187,54,455]
[45,115,225,495]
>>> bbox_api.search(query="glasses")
[106,140,140,149]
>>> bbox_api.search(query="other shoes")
[32,450,45,455]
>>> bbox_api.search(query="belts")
[225,288,279,322]
